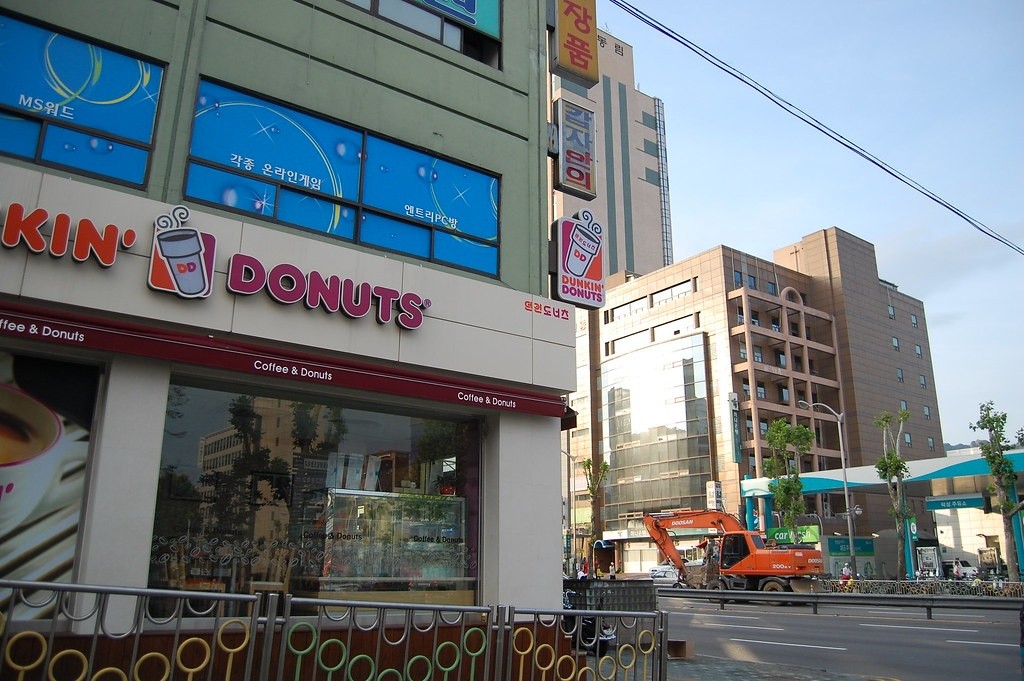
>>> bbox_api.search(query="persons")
[578,557,586,579]
[595,559,603,577]
[840,562,854,592]
[954,562,960,586]
[609,562,616,579]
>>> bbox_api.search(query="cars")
[650,557,691,588]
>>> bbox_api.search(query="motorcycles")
[562,589,617,658]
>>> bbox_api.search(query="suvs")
[941,559,979,580]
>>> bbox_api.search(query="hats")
[611,562,614,565]
[845,563,848,566]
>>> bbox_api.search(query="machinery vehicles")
[642,506,830,607]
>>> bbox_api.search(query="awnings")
[594,540,615,548]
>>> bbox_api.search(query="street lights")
[797,399,864,581]
